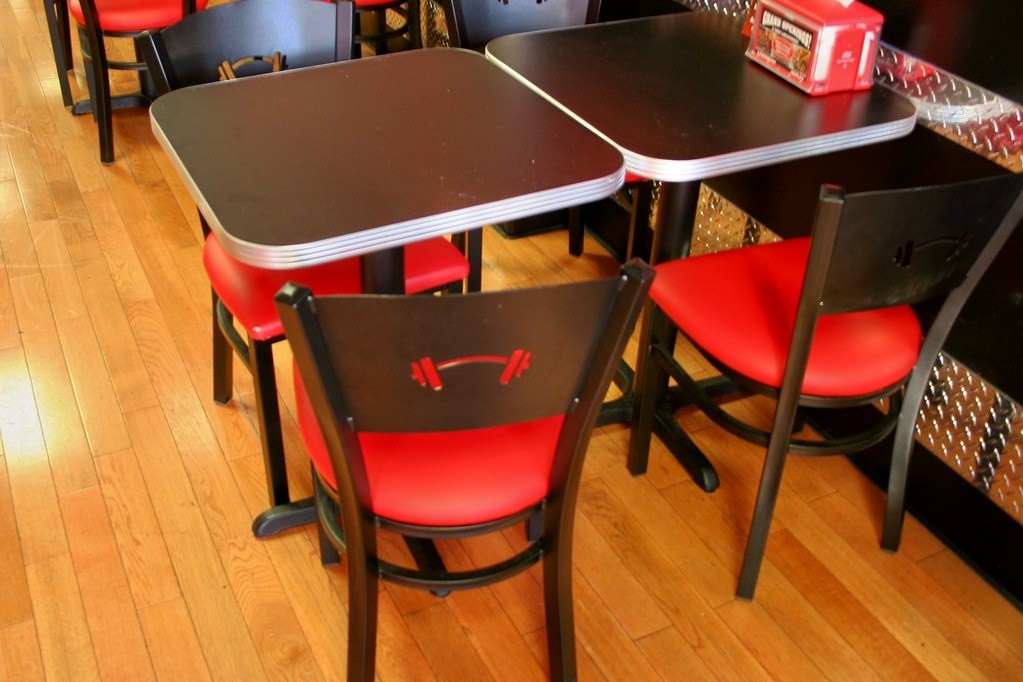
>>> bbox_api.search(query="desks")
[145,46,628,541]
[486,12,917,490]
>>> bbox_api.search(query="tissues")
[744,0,884,97]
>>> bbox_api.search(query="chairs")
[42,0,1023,682]
